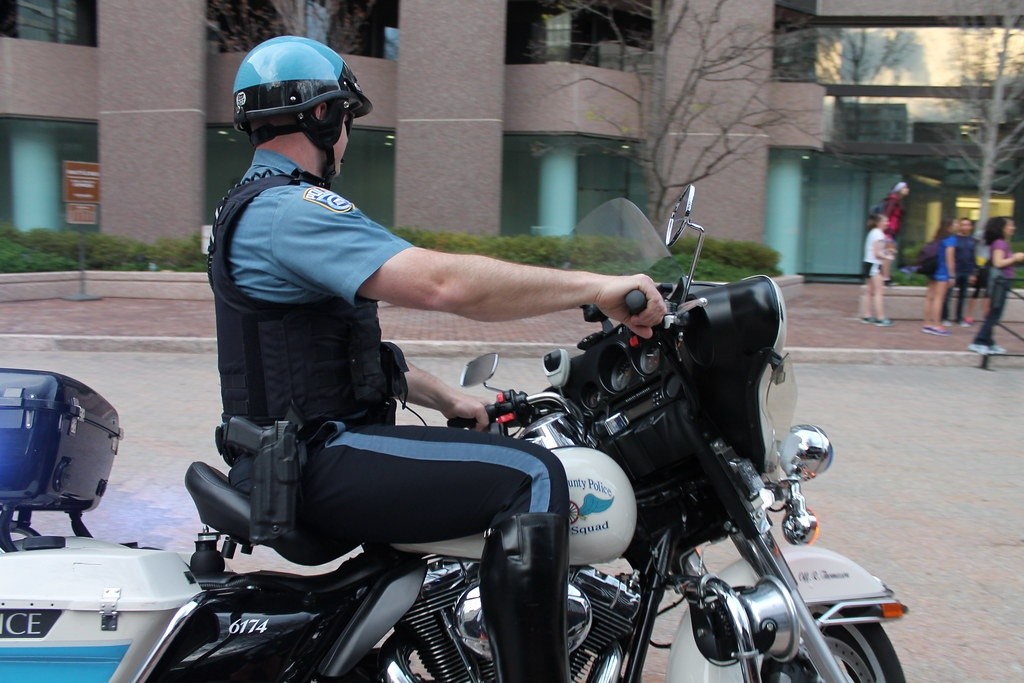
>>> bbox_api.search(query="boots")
[478,512,572,683]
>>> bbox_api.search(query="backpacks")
[911,239,939,275]
[972,239,1005,301]
[868,202,885,218]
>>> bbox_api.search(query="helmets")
[233,36,374,133]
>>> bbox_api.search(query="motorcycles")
[0,181,913,683]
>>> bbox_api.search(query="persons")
[968,216,1024,355]
[921,219,960,338]
[207,36,668,683]
[942,218,981,328]
[874,181,910,285]
[859,213,897,326]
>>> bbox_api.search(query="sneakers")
[921,323,951,337]
[968,343,1007,356]
[861,316,896,327]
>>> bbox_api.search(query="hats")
[892,180,907,192]
